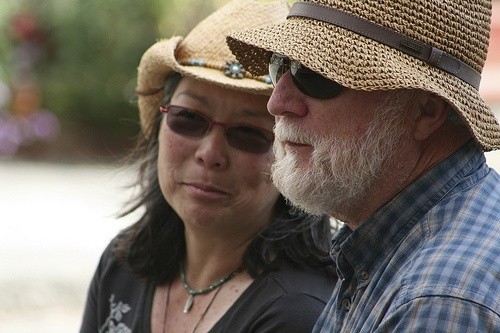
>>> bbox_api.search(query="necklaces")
[162,257,245,333]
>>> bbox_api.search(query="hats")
[135,0,298,145]
[226,0,500,152]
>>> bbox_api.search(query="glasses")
[156,102,275,154]
[269,50,344,100]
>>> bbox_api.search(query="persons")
[78,0,336,333]
[224,0,500,333]
